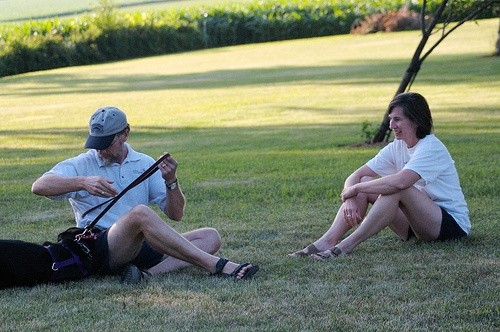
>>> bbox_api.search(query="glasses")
[105,134,121,149]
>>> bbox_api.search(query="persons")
[288,93,471,260]
[31,107,260,285]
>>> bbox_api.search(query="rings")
[346,214,351,217]
[162,164,166,168]
[101,191,105,195]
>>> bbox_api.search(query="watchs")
[165,178,178,190]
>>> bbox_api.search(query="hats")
[83,107,127,149]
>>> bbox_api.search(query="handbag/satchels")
[56,225,99,277]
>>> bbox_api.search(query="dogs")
[0,227,93,290]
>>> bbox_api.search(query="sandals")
[285,244,322,259]
[310,247,342,263]
[209,258,259,285]
[120,264,154,286]
